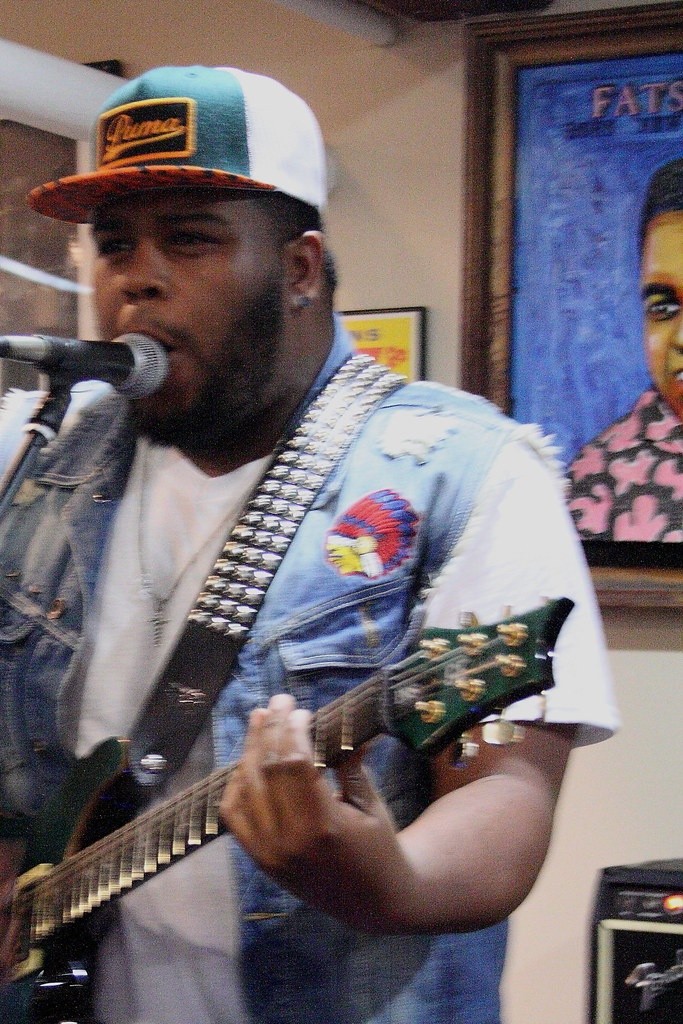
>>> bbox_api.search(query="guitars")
[0,596,577,1024]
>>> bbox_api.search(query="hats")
[24,65,329,215]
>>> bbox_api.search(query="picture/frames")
[458,2,682,608]
[336,305,427,382]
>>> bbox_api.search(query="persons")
[0,66,621,1024]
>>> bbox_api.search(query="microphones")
[0,330,170,400]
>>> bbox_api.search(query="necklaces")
[139,428,283,648]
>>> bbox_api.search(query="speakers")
[587,860,683,1024]
